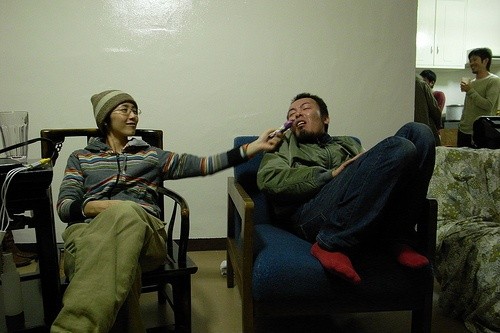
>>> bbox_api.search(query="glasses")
[112,109,142,115]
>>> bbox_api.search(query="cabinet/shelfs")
[416,0,468,70]
[466,1,499,64]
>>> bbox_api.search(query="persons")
[257,93,437,286]
[50,89,285,333]
[415,70,445,146]
[457,47,500,148]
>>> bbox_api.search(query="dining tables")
[0,158,62,332]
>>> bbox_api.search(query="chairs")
[40,129,198,333]
[226,134,438,333]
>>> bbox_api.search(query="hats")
[91,90,138,130]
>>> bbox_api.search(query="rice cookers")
[446,104,464,122]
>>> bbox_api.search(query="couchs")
[425,145,500,332]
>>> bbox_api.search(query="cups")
[0,111,29,160]
[461,77,470,92]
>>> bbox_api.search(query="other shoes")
[6,245,36,260]
[1,251,31,268]
